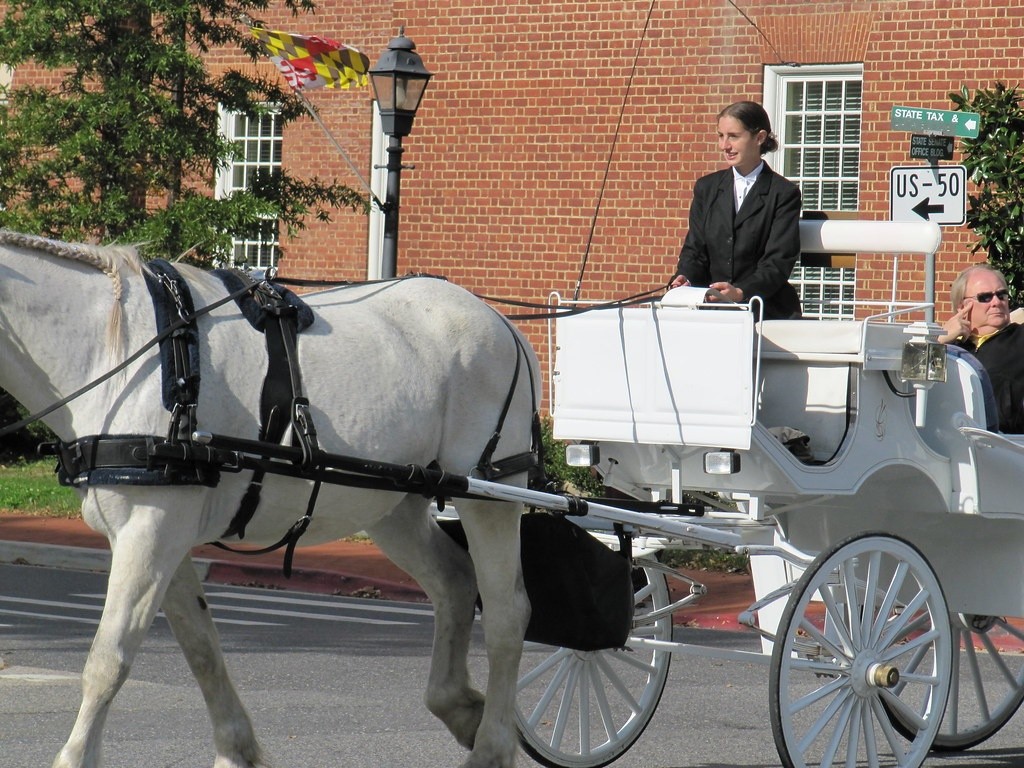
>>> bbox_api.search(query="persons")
[667,101,802,320]
[938,264,1024,435]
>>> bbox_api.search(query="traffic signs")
[889,165,968,227]
[890,105,981,139]
[909,134,954,162]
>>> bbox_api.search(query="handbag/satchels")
[436,506,633,652]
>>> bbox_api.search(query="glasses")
[963,288,1009,303]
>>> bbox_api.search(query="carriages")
[1,225,1024,768]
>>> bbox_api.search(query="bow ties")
[735,172,757,182]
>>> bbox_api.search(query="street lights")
[369,23,436,283]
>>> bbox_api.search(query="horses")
[0,234,543,768]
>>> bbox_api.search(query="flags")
[252,25,370,92]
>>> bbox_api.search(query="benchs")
[909,344,1024,458]
[753,219,943,363]
